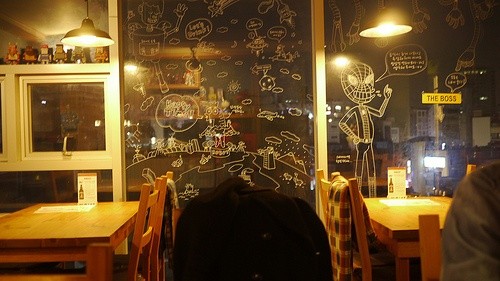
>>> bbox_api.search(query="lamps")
[60,0,115,47]
[358,0,413,38]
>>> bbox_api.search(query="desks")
[367,196,453,281]
[0,199,139,281]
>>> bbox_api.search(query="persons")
[439,161,500,281]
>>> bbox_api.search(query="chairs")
[317,169,442,281]
[113,171,173,281]
